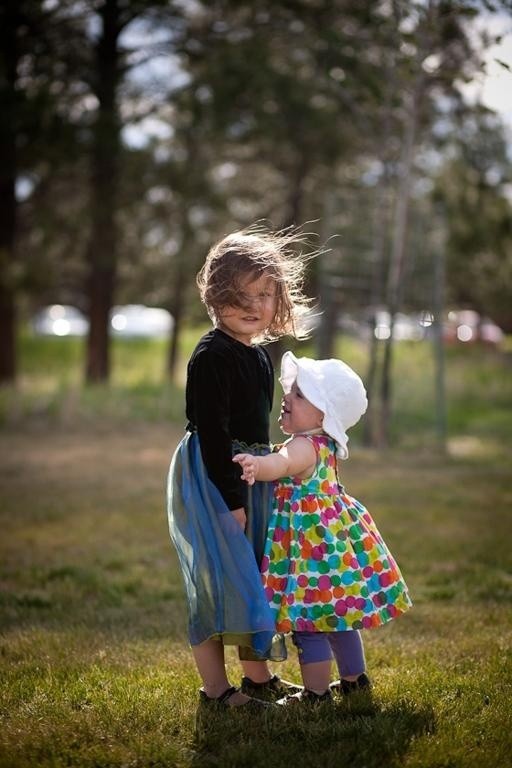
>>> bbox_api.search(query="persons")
[226,349,414,713]
[166,216,339,718]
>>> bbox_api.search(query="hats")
[278,350,368,462]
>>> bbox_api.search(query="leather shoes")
[329,671,370,695]
[198,685,276,714]
[242,674,302,703]
[275,687,333,708]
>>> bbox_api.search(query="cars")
[373,311,425,344]
[34,304,88,340]
[336,314,372,344]
[443,309,503,348]
[109,302,175,338]
